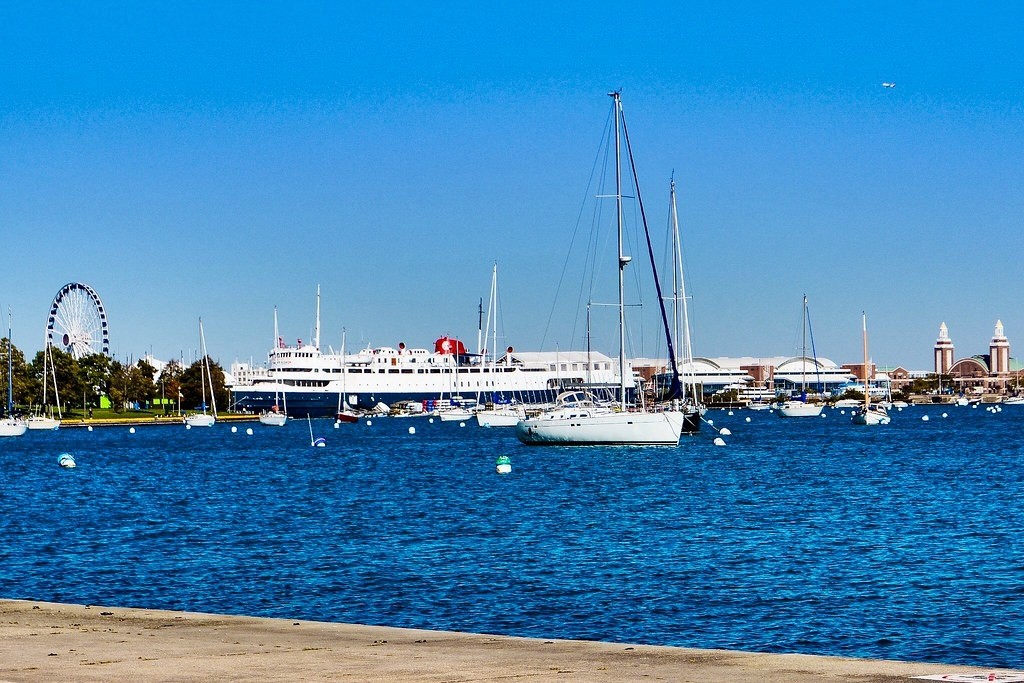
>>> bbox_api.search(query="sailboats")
[25,325,62,429]
[518,86,705,447]
[0,308,29,437]
[341,259,527,427]
[775,292,828,417]
[259,305,288,426]
[852,311,891,426]
[185,317,217,427]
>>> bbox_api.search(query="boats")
[233,282,634,418]
